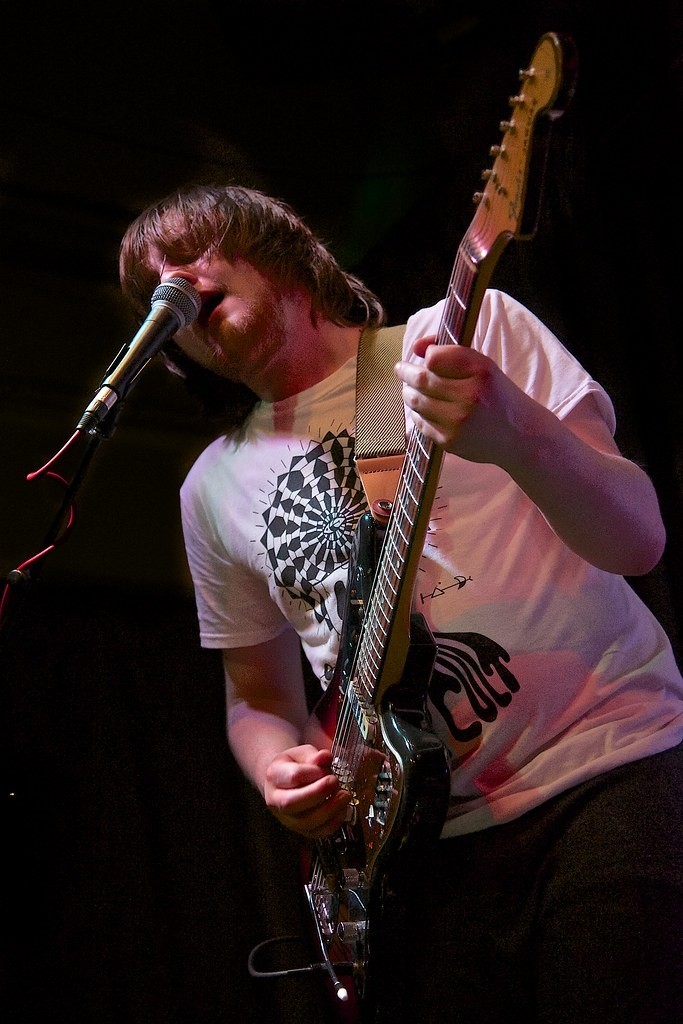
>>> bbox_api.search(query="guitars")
[297,32,576,1024]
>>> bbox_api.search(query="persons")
[116,184,682,1024]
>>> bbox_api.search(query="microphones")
[77,276,202,433]
